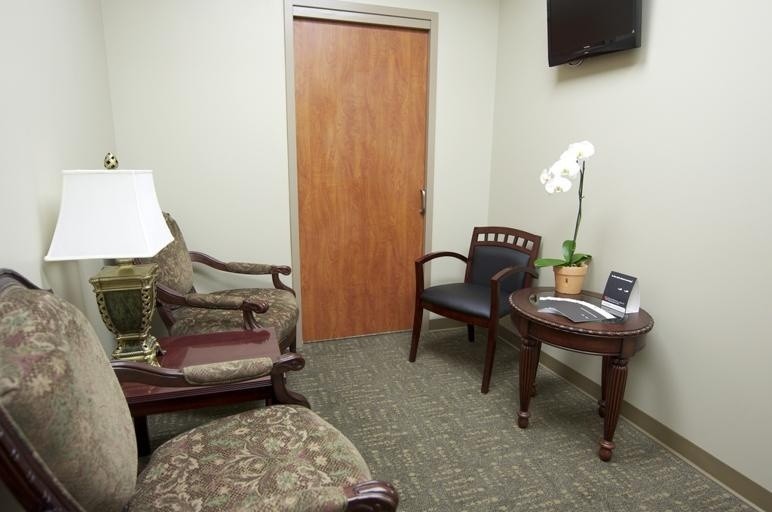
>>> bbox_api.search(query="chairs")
[0,269,402,512]
[409,226,542,394]
[134,209,299,354]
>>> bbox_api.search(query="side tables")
[109,323,287,455]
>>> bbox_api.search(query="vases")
[553,264,588,295]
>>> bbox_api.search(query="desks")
[508,286,655,461]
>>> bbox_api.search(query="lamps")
[42,152,168,367]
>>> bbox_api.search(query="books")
[535,295,617,324]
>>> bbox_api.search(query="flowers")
[533,140,592,268]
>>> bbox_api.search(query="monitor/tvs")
[547,0,642,67]
